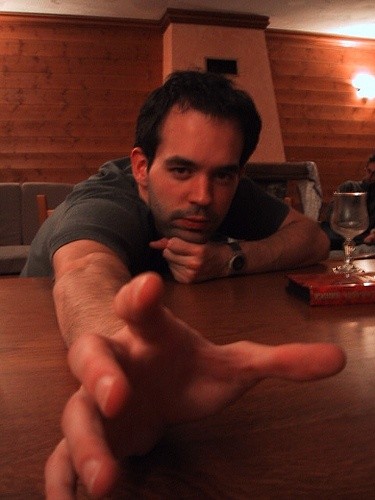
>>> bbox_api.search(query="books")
[289,269,375,307]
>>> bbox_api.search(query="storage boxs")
[284,275,375,307]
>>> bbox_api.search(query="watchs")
[225,236,247,277]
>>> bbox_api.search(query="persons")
[20,65,348,500]
[327,154,375,249]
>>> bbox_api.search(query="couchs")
[0,162,326,277]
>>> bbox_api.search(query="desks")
[0,254,375,500]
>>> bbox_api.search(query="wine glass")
[331,192,368,274]
